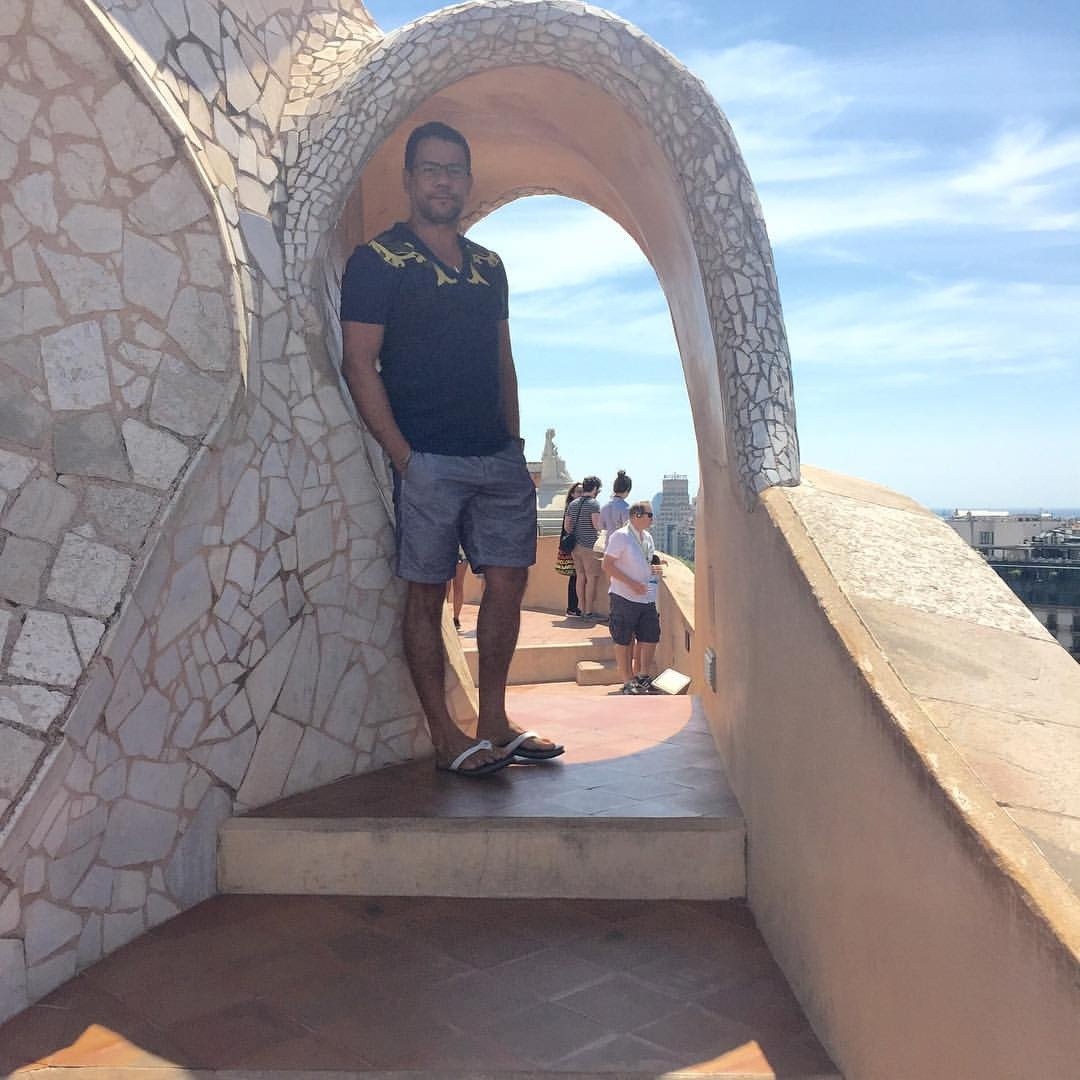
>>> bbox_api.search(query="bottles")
[648,576,656,600]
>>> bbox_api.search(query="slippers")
[435,739,515,778]
[501,731,566,760]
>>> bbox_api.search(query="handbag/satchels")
[560,531,576,553]
[592,529,608,553]
[555,550,576,576]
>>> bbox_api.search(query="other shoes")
[566,608,584,618]
[624,685,645,695]
[632,676,639,689]
[579,609,609,623]
[636,676,657,691]
[454,618,464,632]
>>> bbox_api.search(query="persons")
[445,544,468,628]
[598,471,633,544]
[560,482,581,618]
[602,500,661,694]
[340,121,565,778]
[564,476,603,621]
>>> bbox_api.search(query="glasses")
[408,161,470,180]
[632,511,653,518]
